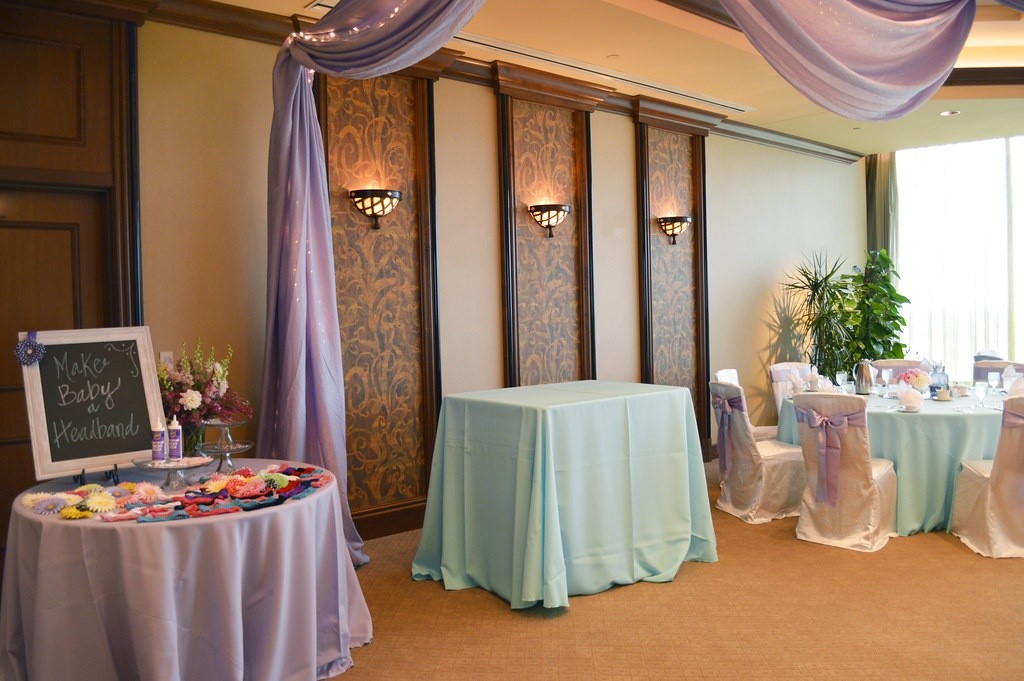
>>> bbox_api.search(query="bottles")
[150,417,166,463]
[168,414,183,461]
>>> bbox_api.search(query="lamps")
[350,189,401,230]
[530,202,570,237]
[660,216,693,244]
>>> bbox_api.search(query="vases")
[900,385,924,411]
[183,415,206,454]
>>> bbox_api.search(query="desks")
[776,387,1011,539]
[411,378,717,609]
[0,458,374,680]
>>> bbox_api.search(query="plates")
[806,388,818,392]
[898,405,920,412]
[933,396,953,401]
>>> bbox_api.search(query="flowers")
[158,334,257,427]
[895,368,933,389]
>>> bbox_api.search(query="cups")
[810,380,818,389]
[936,389,950,399]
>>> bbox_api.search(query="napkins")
[808,364,818,380]
[1003,364,1016,381]
[790,368,802,383]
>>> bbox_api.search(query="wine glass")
[882,368,893,388]
[975,381,988,407]
[836,372,848,393]
[988,372,1000,395]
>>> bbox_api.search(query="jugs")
[853,358,875,394]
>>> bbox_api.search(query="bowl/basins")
[1002,373,1023,393]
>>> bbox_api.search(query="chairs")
[949,396,1024,559]
[870,359,927,387]
[974,359,1024,388]
[793,390,900,553]
[770,359,825,445]
[716,368,779,443]
[706,381,806,524]
